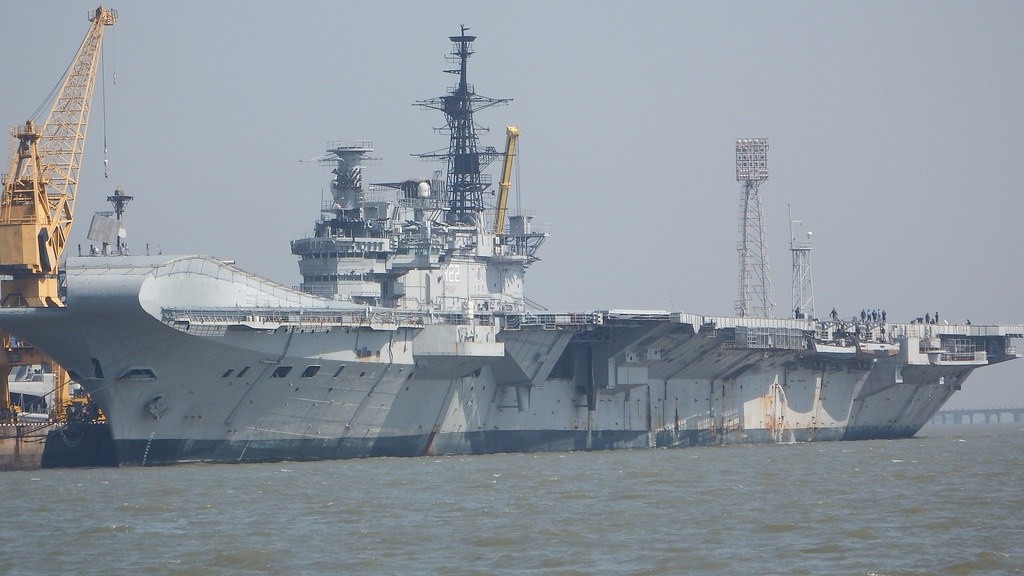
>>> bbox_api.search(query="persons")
[260,314,371,328]
[967,319,971,325]
[795,307,800,319]
[911,312,939,324]
[162,311,247,326]
[860,308,887,322]
[829,308,838,320]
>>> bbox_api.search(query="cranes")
[494,125,523,235]
[0,5,120,427]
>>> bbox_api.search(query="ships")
[0,24,1024,466]
